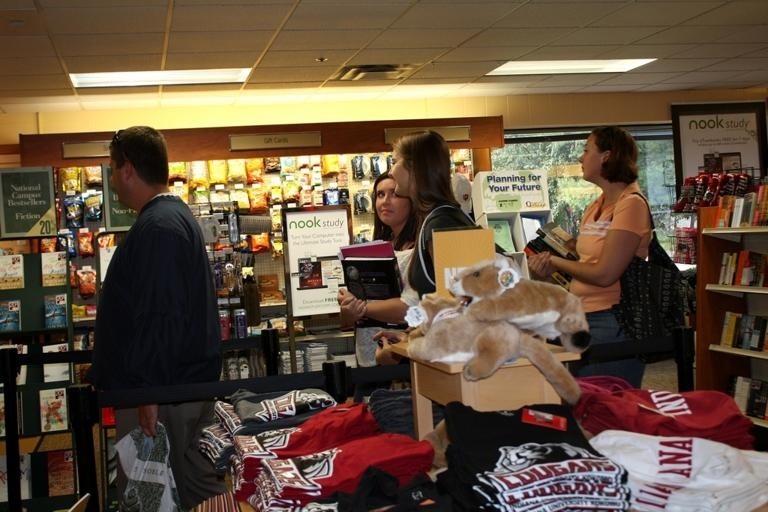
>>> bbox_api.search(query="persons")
[373,129,497,368]
[528,126,652,390]
[337,172,421,367]
[84,126,222,511]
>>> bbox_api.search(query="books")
[712,189,768,423]
[522,223,580,289]
[340,256,408,329]
[0,238,71,437]
[340,240,395,256]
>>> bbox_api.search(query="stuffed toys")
[446,259,592,354]
[402,291,583,405]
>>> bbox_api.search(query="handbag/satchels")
[611,193,696,345]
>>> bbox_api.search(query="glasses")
[112,130,135,168]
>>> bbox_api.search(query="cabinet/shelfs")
[689,220,768,433]
